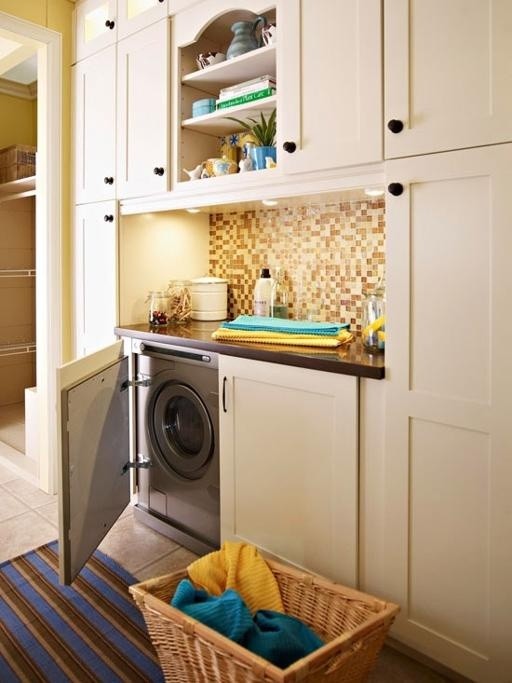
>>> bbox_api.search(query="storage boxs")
[0,143,36,184]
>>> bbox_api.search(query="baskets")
[126,547,407,681]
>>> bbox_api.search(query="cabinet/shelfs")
[71,0,118,202]
[282,0,384,177]
[118,0,173,202]
[55,336,360,593]
[170,0,285,209]
[385,144,512,683]
[381,0,512,160]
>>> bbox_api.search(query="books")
[215,74,276,111]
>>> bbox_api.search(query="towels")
[211,314,355,348]
[217,339,350,358]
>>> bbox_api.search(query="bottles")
[146,289,170,328]
[168,280,193,325]
[309,281,327,322]
[269,260,288,320]
[362,289,386,353]
[226,17,267,61]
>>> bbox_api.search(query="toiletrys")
[269,264,290,322]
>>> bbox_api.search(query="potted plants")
[223,108,276,170]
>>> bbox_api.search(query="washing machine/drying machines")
[61,341,219,587]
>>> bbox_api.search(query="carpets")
[0,538,164,683]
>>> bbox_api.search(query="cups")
[196,51,225,70]
[261,22,276,45]
[212,155,238,176]
[201,158,222,178]
